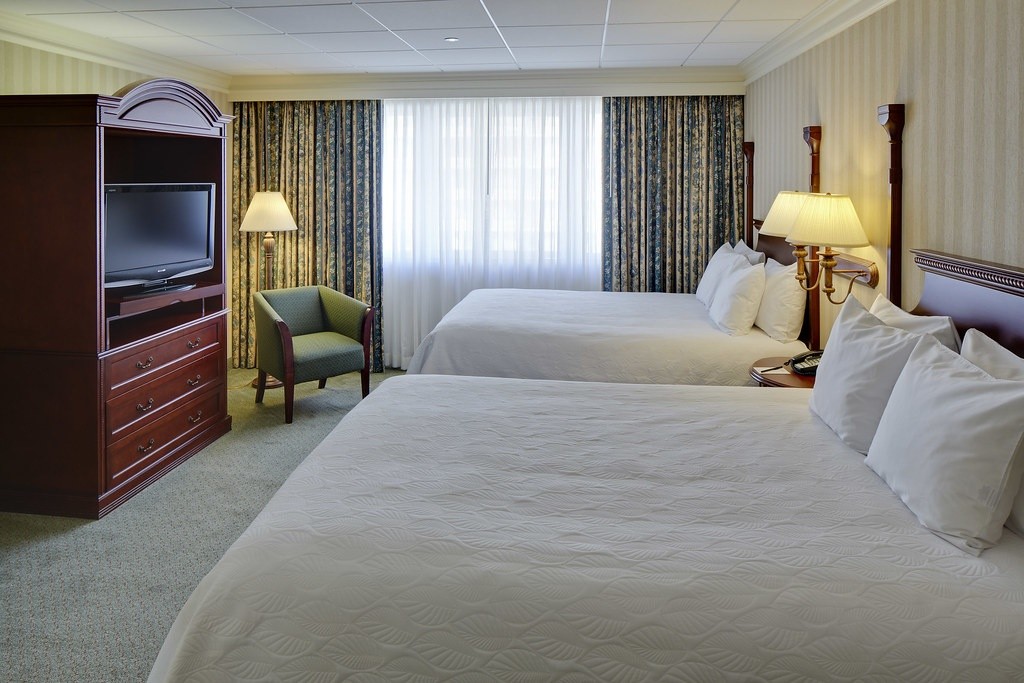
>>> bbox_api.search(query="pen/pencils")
[761,366,783,372]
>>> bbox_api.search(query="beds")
[405,219,820,387]
[146,250,1022,682]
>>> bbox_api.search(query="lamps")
[238,191,299,389]
[759,190,829,289]
[785,193,878,304]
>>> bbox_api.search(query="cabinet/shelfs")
[0,78,237,520]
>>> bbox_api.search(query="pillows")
[695,242,755,311]
[806,292,927,457]
[753,257,807,345]
[707,253,765,338]
[960,328,1022,383]
[869,292,961,354]
[733,239,766,266]
[863,332,1024,557]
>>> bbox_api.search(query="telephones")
[790,350,824,376]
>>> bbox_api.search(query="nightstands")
[748,356,816,388]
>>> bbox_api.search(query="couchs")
[253,285,375,423]
